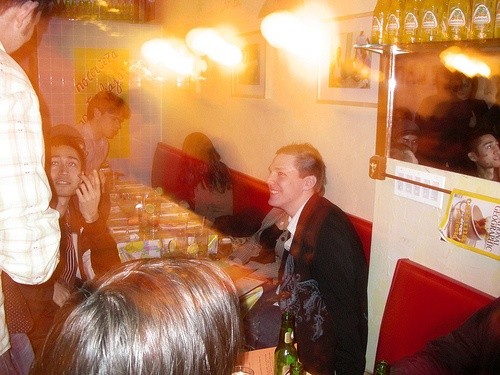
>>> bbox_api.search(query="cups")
[232,365,255,375]
[114,183,208,259]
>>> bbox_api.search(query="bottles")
[273,312,305,375]
[372,0,500,43]
[375,361,389,375]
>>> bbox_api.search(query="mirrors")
[387,38,500,183]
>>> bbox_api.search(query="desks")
[106,178,238,264]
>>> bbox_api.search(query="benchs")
[373,259,500,375]
[150,144,372,265]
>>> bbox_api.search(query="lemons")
[145,204,154,213]
[187,243,199,256]
[155,187,164,196]
[168,240,176,252]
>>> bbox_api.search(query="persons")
[393,62,500,182]
[17,135,122,367]
[32,256,240,375]
[46,87,132,287]
[232,143,368,375]
[178,132,235,224]
[0,0,61,375]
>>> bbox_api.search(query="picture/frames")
[313,12,380,107]
[230,30,265,99]
